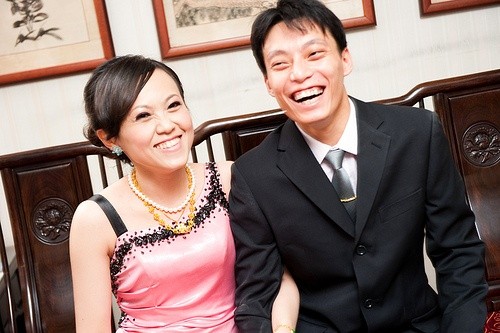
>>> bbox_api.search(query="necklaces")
[126,163,197,234]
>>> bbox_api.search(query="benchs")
[1,68,500,333]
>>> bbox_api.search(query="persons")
[227,0,489,333]
[69,55,300,333]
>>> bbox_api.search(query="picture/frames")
[1,0,117,89]
[150,0,377,61]
[418,0,500,19]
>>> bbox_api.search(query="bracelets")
[274,325,295,333]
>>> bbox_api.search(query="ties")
[325,147,358,225]
